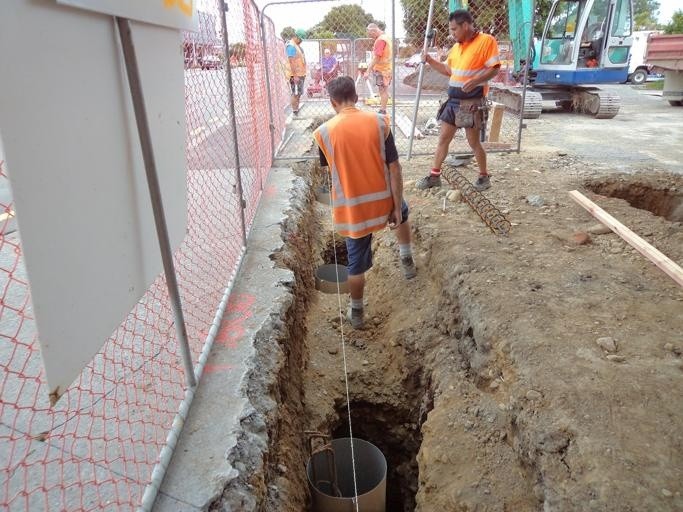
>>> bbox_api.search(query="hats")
[324,49,330,53]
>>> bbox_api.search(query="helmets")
[295,28,306,40]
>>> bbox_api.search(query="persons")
[283,28,310,121]
[415,9,504,191]
[361,23,393,115]
[314,49,338,100]
[312,74,421,330]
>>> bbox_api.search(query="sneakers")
[292,111,308,119]
[475,175,494,193]
[401,255,418,279]
[345,308,365,329]
[413,175,443,189]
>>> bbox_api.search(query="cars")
[405,52,437,67]
[200,56,221,70]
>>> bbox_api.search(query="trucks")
[627,30,683,106]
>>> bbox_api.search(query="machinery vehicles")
[448,0,634,119]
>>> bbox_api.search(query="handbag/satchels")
[375,71,384,86]
[454,102,476,129]
